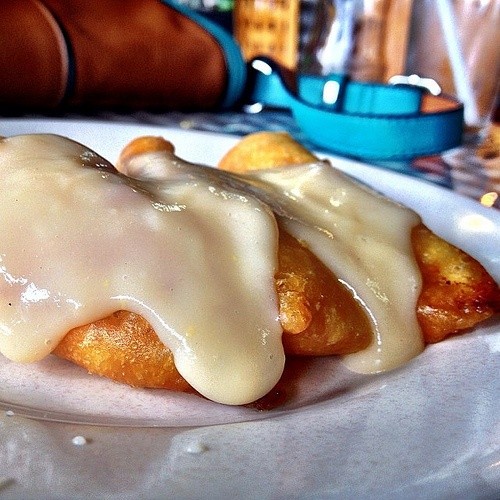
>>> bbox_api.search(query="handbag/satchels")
[1,0,246,111]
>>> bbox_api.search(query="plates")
[0,117,499,500]
